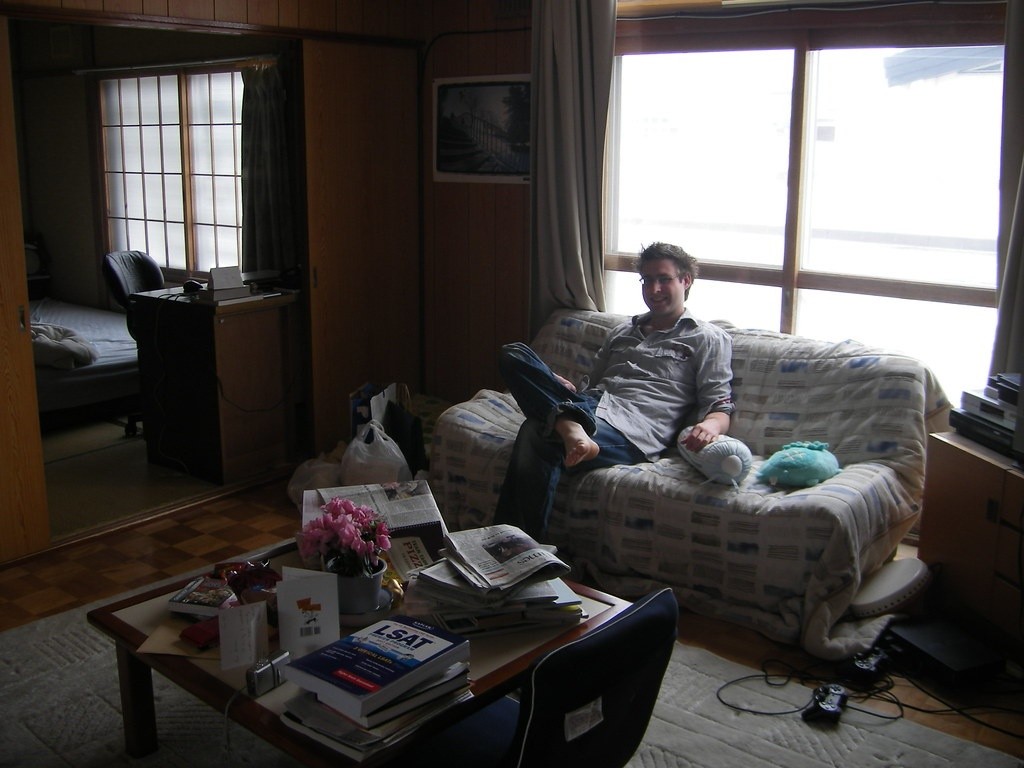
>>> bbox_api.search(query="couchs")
[426,308,929,661]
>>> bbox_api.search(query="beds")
[27,298,139,411]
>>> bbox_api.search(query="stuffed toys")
[756,441,844,489]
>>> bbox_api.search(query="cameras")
[246,649,290,698]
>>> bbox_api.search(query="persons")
[492,242,738,545]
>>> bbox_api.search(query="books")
[167,544,582,767]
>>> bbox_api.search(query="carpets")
[0,538,1024,768]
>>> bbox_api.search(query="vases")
[326,556,386,612]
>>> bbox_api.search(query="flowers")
[295,493,392,578]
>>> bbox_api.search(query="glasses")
[639,272,683,285]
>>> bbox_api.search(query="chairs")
[102,251,166,434]
[394,588,679,768]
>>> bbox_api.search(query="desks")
[85,541,636,768]
[130,285,304,485]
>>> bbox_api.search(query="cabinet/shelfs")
[917,432,1024,667]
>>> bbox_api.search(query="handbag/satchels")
[350,381,397,441]
[384,382,422,480]
[287,459,339,513]
[340,419,412,484]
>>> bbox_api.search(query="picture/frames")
[431,76,534,184]
